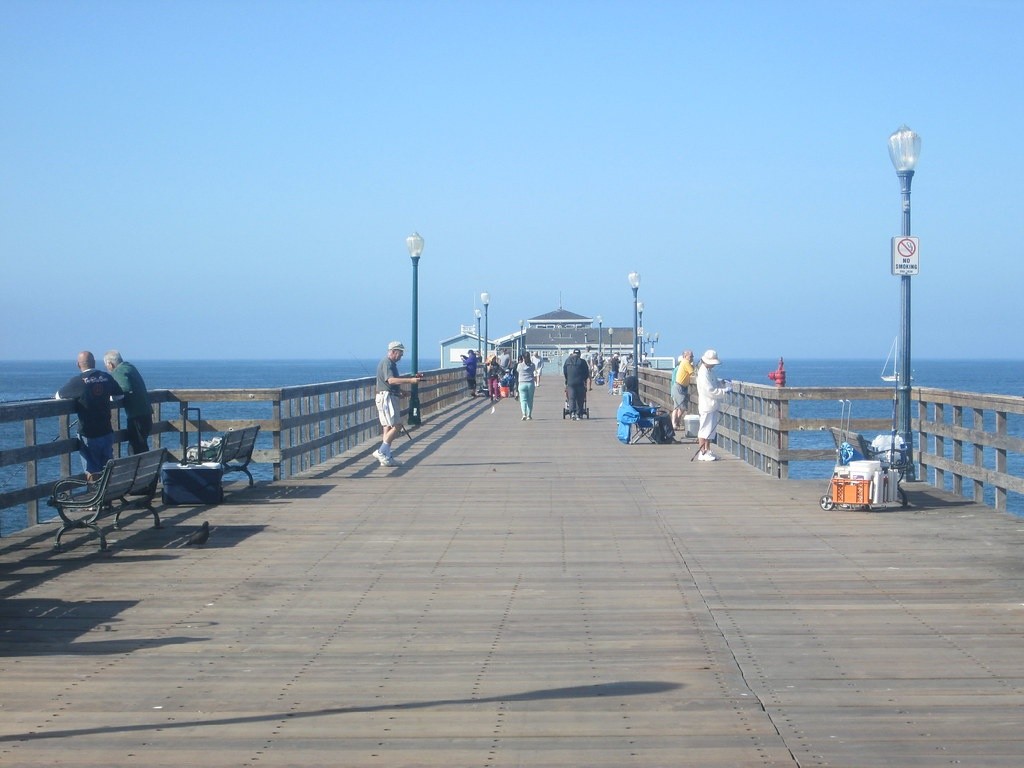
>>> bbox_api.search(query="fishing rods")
[691,367,757,461]
[668,355,732,415]
[416,372,492,382]
[350,351,413,440]
[0,419,78,488]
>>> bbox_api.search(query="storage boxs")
[159,460,224,504]
[833,477,872,505]
[683,414,701,437]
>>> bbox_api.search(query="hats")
[573,349,580,355]
[389,342,405,350]
[702,349,720,365]
[678,356,683,362]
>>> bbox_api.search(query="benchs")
[830,427,908,508]
[47,447,167,552]
[183,424,262,501]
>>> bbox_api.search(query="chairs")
[622,392,657,444]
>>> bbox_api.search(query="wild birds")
[490,407,495,414]
[187,521,209,546]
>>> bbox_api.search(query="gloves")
[723,387,732,393]
[722,379,731,385]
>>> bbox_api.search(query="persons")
[516,352,536,420]
[622,376,681,444]
[618,352,633,396]
[694,350,733,460]
[372,341,421,467]
[587,360,592,390]
[460,350,477,397]
[639,351,650,367]
[563,349,589,420]
[608,352,621,394]
[670,349,696,430]
[483,354,505,404]
[592,351,606,379]
[531,351,544,386]
[103,350,154,494]
[55,351,125,511]
[498,350,523,401]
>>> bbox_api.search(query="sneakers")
[381,458,402,466]
[698,449,716,461]
[373,450,391,466]
[707,449,720,460]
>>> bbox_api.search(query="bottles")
[873,469,898,504]
[649,402,655,413]
[833,465,848,485]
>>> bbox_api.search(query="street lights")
[636,301,644,362]
[596,314,603,354]
[475,309,482,356]
[627,270,641,377]
[481,291,490,389]
[646,331,660,357]
[886,122,923,482]
[519,318,526,356]
[406,231,425,425]
[608,327,616,357]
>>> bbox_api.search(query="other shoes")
[521,416,526,421]
[672,425,684,430]
[665,438,681,443]
[527,416,532,420]
[656,439,666,444]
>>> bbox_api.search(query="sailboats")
[881,336,914,382]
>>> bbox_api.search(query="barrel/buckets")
[849,460,881,499]
[500,387,510,398]
